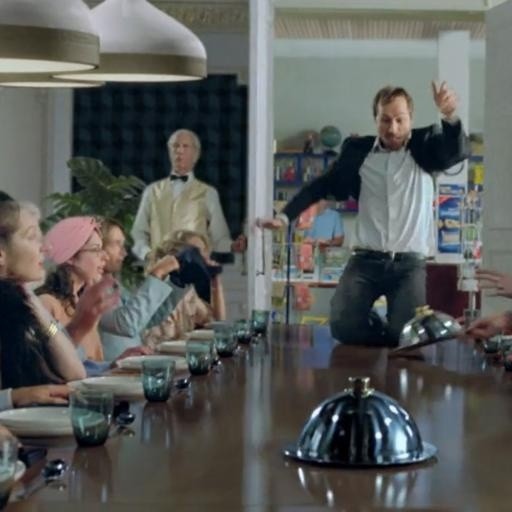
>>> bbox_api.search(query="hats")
[47,215,104,265]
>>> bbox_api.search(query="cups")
[140,358,176,404]
[138,402,174,449]
[0,436,21,510]
[462,309,511,373]
[232,319,253,346]
[214,325,240,358]
[239,336,267,368]
[184,335,215,378]
[66,442,114,503]
[66,388,115,450]
[251,309,269,334]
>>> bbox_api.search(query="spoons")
[12,455,67,504]
[170,375,191,400]
[107,410,136,437]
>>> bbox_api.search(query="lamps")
[0,0,210,91]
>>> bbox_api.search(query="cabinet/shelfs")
[273,152,358,213]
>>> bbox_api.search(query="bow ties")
[171,174,188,182]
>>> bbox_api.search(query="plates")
[117,355,188,368]
[0,408,103,438]
[65,374,142,396]
[184,320,235,339]
[155,340,184,354]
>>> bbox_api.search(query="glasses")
[79,247,103,254]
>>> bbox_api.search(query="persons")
[456,265,511,338]
[142,228,224,349]
[0,189,84,412]
[33,216,154,374]
[301,196,344,247]
[90,215,179,363]
[132,129,231,269]
[254,79,471,350]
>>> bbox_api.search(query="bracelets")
[44,321,58,338]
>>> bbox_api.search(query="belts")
[354,249,425,262]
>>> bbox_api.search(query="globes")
[319,124,343,157]
